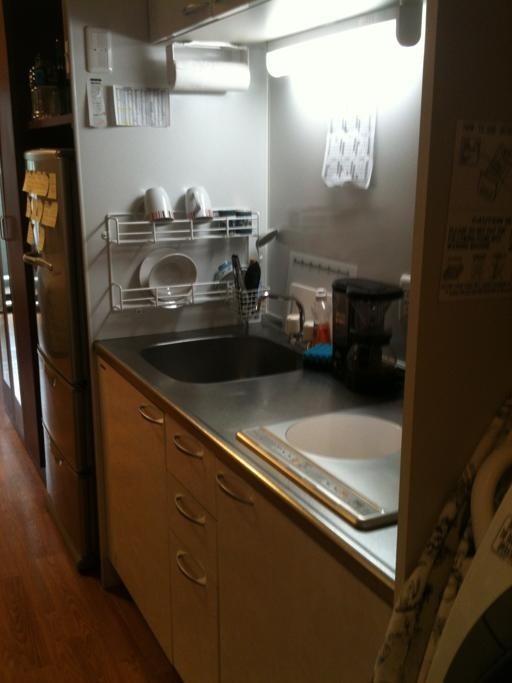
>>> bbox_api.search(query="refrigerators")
[17,145,97,578]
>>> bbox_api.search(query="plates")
[139,246,197,301]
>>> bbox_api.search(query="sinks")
[139,334,311,384]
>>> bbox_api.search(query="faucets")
[254,290,310,350]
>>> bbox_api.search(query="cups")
[143,186,175,226]
[185,185,213,222]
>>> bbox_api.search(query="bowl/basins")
[213,261,248,297]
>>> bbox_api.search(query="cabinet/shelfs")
[96,358,393,682]
[100,209,270,320]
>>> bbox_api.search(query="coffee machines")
[329,277,407,403]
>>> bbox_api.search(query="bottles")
[311,288,332,348]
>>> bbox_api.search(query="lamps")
[261,0,424,77]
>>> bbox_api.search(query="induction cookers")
[235,400,398,532]
